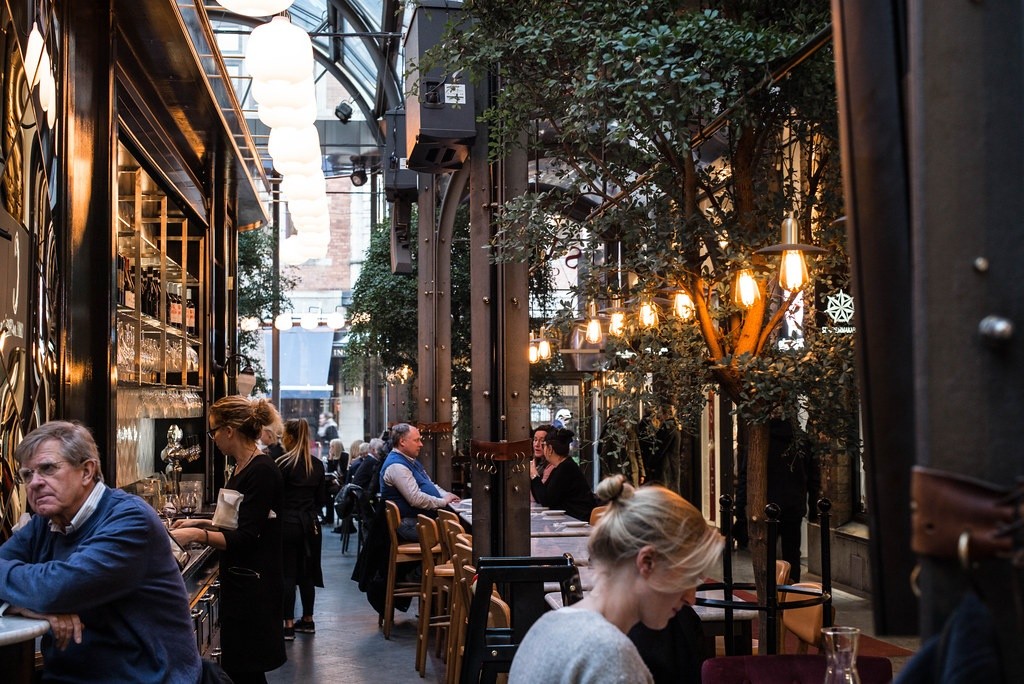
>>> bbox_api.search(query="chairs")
[383,499,510,684]
[712,561,840,670]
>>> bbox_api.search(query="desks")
[446,498,761,655]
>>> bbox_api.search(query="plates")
[562,522,588,526]
[530,506,549,511]
[544,509,566,514]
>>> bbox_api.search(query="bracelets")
[201,529,208,547]
[531,475,540,480]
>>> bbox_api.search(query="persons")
[380,424,460,543]
[508,475,725,684]
[598,405,682,495]
[262,419,327,641]
[173,394,287,684]
[316,412,338,445]
[529,424,595,523]
[322,430,393,535]
[0,422,202,684]
[766,413,822,582]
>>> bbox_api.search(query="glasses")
[541,442,549,450]
[207,425,235,440]
[14,460,75,485]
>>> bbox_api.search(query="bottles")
[819,625,863,684]
[115,251,198,335]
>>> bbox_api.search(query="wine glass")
[135,471,198,522]
[112,320,206,419]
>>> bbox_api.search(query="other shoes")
[319,515,334,526]
[294,619,315,633]
[283,626,295,640]
[331,526,357,533]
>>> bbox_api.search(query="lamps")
[212,0,335,270]
[524,205,836,364]
